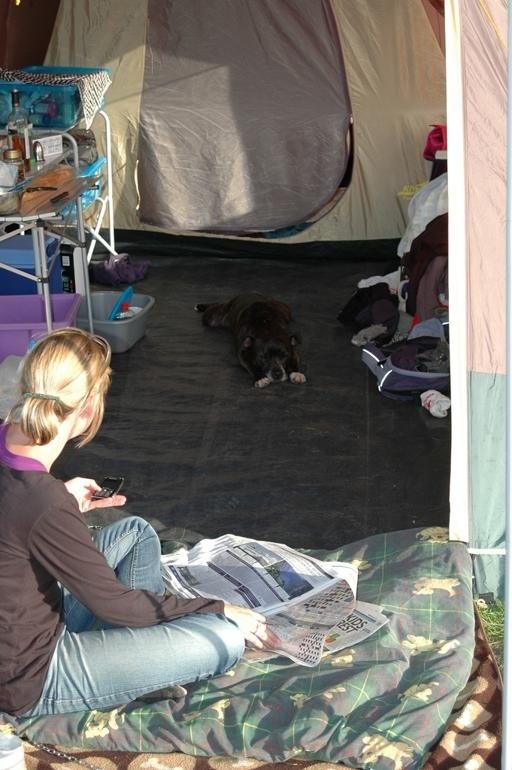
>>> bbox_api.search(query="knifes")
[36,191,68,212]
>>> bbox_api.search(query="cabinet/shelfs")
[0,111,122,339]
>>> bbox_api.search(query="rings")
[253,624,260,634]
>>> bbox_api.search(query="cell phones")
[93,475,123,503]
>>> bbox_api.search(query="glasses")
[41,327,112,379]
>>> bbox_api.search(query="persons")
[0,327,282,717]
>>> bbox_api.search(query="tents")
[42,0,445,266]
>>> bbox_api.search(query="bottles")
[0,88,30,184]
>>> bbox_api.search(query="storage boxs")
[0,292,83,363]
[0,234,63,296]
[75,290,156,353]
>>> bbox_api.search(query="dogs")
[194,295,308,388]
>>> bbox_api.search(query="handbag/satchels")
[336,283,450,399]
[423,125,447,161]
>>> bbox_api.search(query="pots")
[0,184,57,215]
